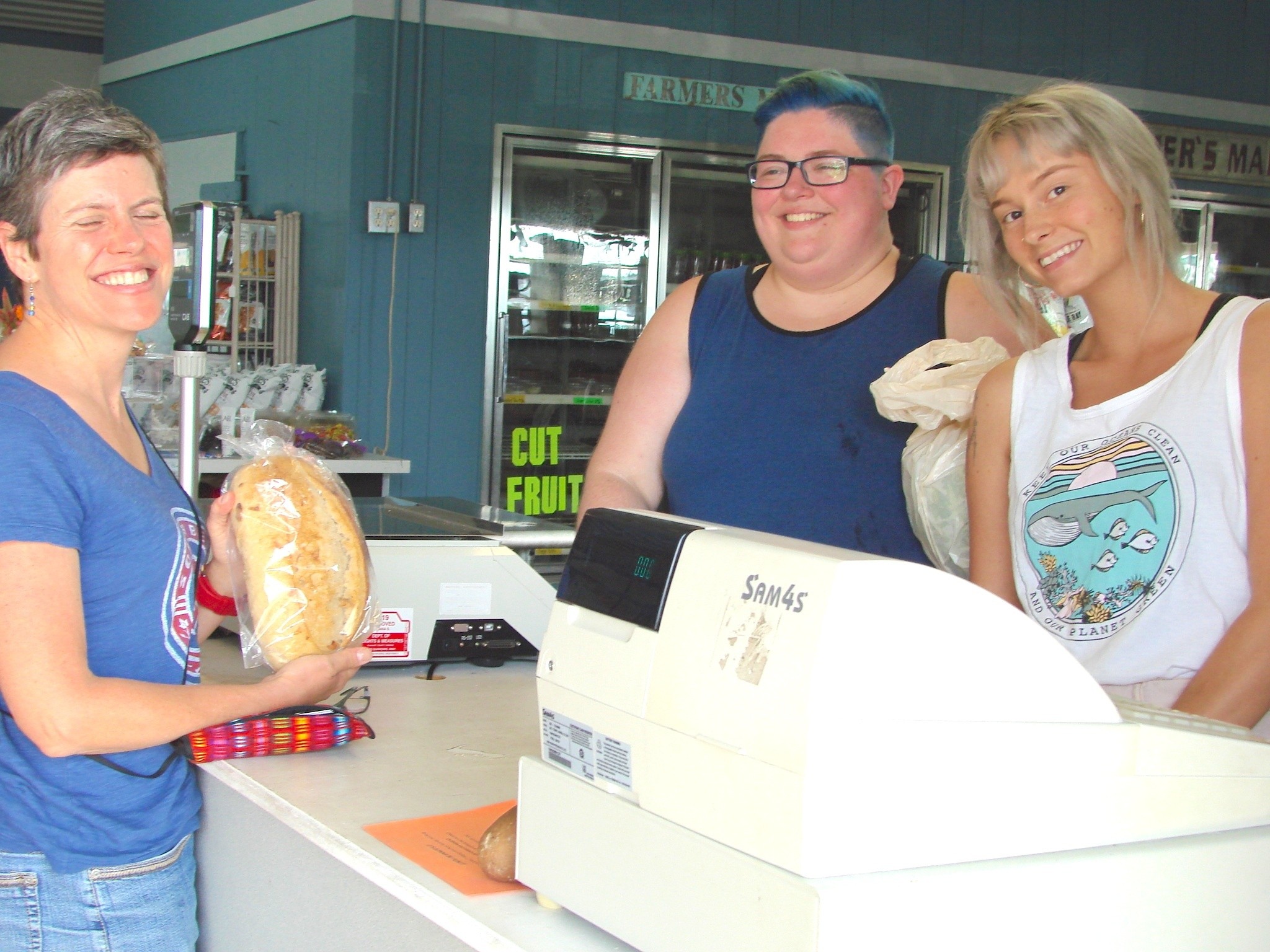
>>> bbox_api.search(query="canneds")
[668,247,768,284]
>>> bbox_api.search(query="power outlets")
[408,203,426,234]
[367,200,400,233]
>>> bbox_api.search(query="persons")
[578,66,1060,580]
[0,88,370,951]
[968,84,1270,736]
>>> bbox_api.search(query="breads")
[478,806,517,884]
[229,451,369,668]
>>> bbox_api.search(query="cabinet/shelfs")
[204,212,299,364]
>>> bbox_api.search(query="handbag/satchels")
[870,337,1013,582]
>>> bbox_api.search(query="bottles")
[668,245,769,283]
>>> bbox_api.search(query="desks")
[199,453,410,496]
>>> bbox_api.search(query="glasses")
[748,156,892,189]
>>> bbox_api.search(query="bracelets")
[195,572,248,616]
